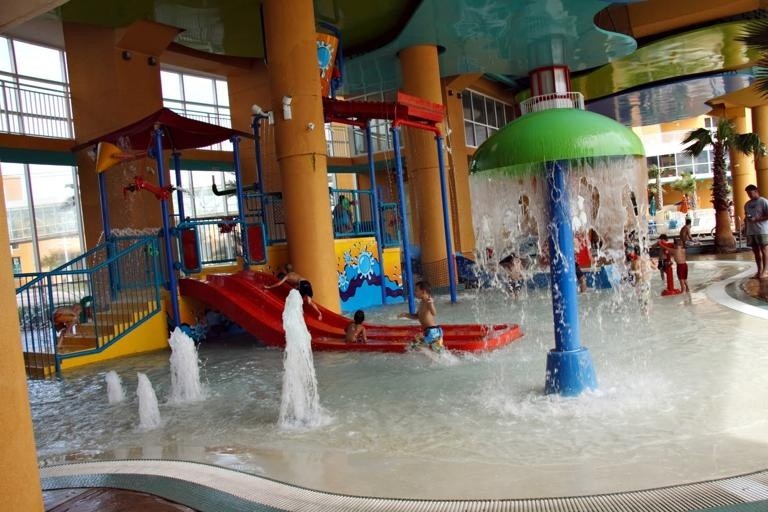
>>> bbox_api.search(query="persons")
[53,303,83,348]
[502,194,702,296]
[400,281,443,350]
[344,311,369,343]
[332,195,353,238]
[744,185,768,279]
[263,271,322,321]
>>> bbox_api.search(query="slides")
[181,271,525,351]
[402,245,621,288]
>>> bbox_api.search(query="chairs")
[645,207,747,247]
